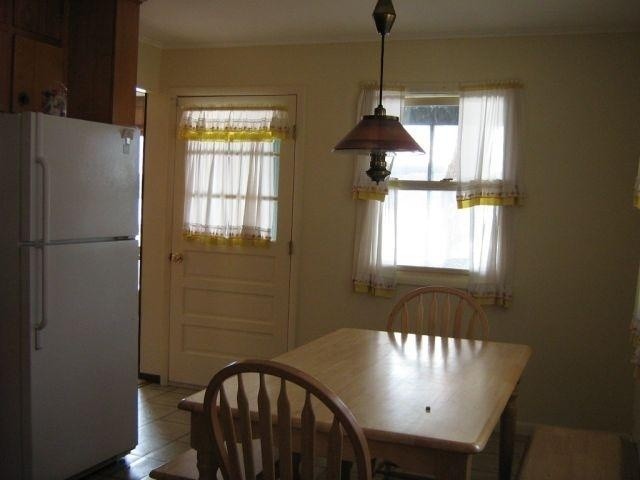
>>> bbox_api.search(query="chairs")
[385,285,491,344]
[203,357,376,480]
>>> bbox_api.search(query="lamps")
[329,0,427,186]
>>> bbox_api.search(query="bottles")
[41,79,67,116]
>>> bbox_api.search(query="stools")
[148,439,287,480]
[515,424,625,480]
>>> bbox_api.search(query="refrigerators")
[0,110,140,479]
